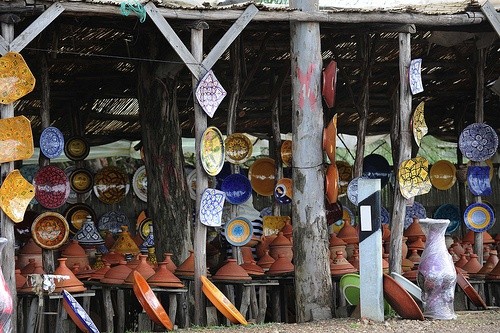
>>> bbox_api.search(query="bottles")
[418,217,456,322]
[0,238,18,333]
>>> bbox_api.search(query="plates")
[198,275,248,325]
[339,272,391,315]
[59,290,102,333]
[61,166,93,205]
[64,137,90,161]
[64,204,97,236]
[133,270,174,331]
[40,127,64,159]
[0,51,36,105]
[453,269,488,310]
[382,274,425,321]
[70,169,93,193]
[390,272,426,308]
[32,211,70,249]
[97,58,500,245]
[0,168,34,222]
[0,115,35,164]
[33,166,70,210]
